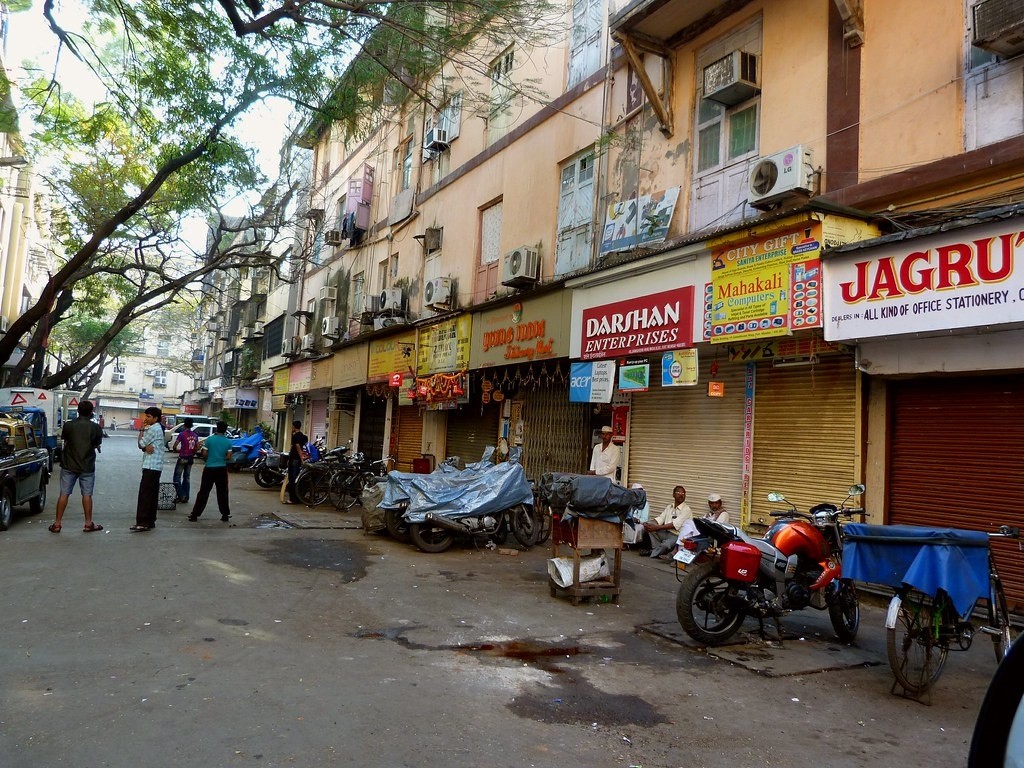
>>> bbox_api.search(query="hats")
[601,425,614,433]
[707,493,721,503]
[631,482,644,490]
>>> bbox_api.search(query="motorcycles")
[673,483,873,649]
[226,435,352,501]
[381,493,540,555]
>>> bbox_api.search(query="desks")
[549,516,624,606]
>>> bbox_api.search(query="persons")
[99,415,104,428]
[188,421,234,523]
[130,417,134,430]
[639,486,693,557]
[130,407,165,532]
[659,493,730,566]
[160,414,167,435]
[48,400,104,533]
[622,483,650,550]
[172,417,199,504]
[302,434,318,464]
[282,421,308,505]
[587,425,619,485]
[111,417,116,431]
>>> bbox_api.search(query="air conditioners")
[325,230,341,245]
[209,316,263,339]
[747,144,814,207]
[971,0,1024,59]
[700,49,761,106]
[379,287,402,313]
[322,316,339,336]
[503,245,538,288]
[425,128,448,150]
[301,335,315,350]
[282,339,298,356]
[424,277,451,307]
[320,286,337,301]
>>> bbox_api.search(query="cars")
[162,422,208,449]
[167,424,234,458]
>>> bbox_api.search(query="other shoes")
[640,549,653,557]
[186,515,197,522]
[657,552,675,562]
[128,523,151,532]
[172,498,188,503]
[220,517,229,522]
[283,500,295,504]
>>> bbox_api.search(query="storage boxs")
[720,541,763,582]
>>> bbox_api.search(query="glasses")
[673,491,687,496]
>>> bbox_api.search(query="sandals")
[82,522,104,532]
[48,522,62,533]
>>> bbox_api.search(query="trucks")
[0,405,58,472]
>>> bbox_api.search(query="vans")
[0,413,38,453]
[174,414,220,426]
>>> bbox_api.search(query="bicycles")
[883,524,1016,705]
[291,451,376,508]
[524,478,555,548]
[326,452,396,513]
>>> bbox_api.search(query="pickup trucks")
[0,449,51,532]
